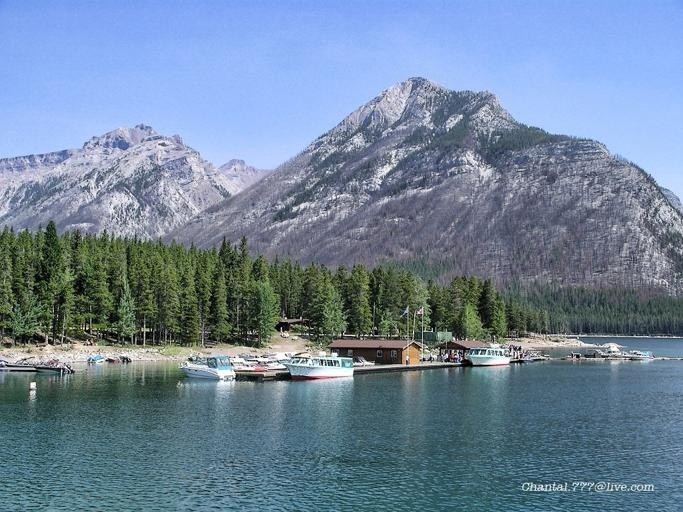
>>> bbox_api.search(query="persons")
[429,350,458,363]
[404,354,409,365]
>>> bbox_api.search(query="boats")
[463,347,510,366]
[512,345,652,363]
[0,353,130,374]
[179,346,355,382]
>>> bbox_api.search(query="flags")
[415,308,422,315]
[399,306,408,317]
